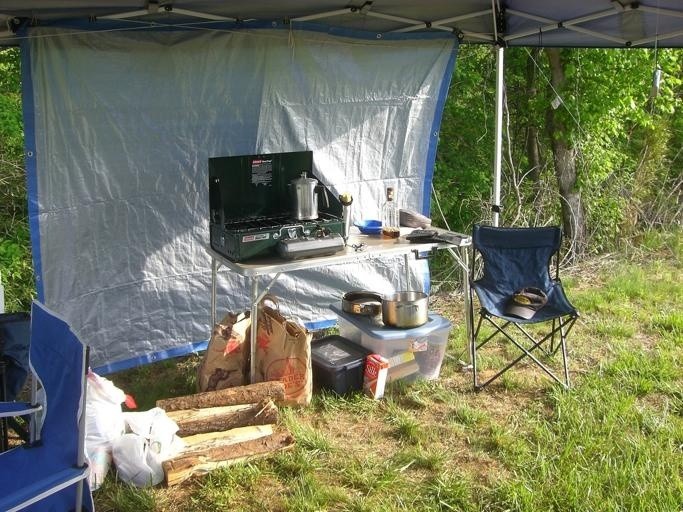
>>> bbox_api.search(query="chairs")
[460,220,581,394]
[0,295,96,511]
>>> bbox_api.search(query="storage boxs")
[309,302,451,393]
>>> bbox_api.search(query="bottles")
[382,188,402,236]
[340,195,351,240]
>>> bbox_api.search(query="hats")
[505,287,549,320]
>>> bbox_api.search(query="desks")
[202,227,474,391]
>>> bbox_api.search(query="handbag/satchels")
[196,294,313,405]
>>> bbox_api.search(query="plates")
[355,218,382,236]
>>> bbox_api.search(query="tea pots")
[287,170,329,222]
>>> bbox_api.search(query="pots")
[342,289,429,328]
